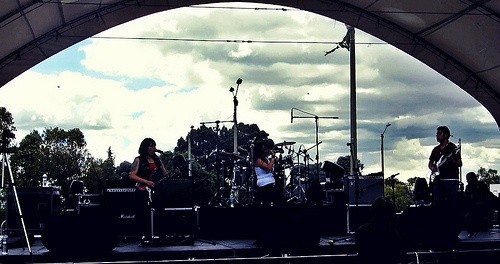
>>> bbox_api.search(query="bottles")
[3,238,6,254]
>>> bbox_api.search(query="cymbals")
[218,141,313,203]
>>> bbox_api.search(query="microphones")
[291,109,293,123]
[154,149,163,154]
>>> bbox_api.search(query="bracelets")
[272,153,275,157]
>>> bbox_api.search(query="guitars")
[430,146,460,183]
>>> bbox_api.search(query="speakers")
[346,176,384,206]
[105,179,149,242]
[162,178,198,207]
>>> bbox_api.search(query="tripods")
[0,147,32,256]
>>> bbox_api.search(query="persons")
[428,125,463,196]
[465,172,492,237]
[355,196,405,264]
[253,130,269,144]
[129,138,166,246]
[253,139,281,206]
